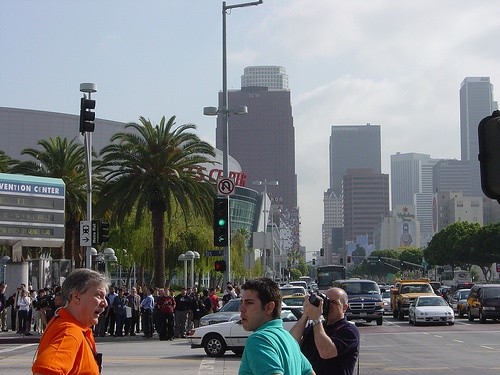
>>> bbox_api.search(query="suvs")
[467,283,500,323]
[198,298,291,325]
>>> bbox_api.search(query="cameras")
[309,292,330,316]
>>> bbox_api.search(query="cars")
[278,286,307,305]
[188,305,325,358]
[409,296,454,325]
[436,286,470,317]
[378,284,393,311]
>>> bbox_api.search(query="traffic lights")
[214,198,228,247]
[400,260,404,266]
[93,219,109,246]
[215,261,225,271]
[80,97,95,133]
[378,256,381,262]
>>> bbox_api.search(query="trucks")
[457,283,475,290]
[430,283,441,292]
[438,270,473,287]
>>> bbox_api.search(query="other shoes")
[0,326,45,337]
[93,330,187,341]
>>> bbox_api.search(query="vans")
[330,277,385,326]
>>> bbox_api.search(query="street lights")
[79,82,97,269]
[178,251,201,289]
[90,248,117,277]
[253,179,282,283]
[202,0,265,290]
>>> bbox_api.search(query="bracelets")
[314,319,322,326]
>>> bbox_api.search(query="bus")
[317,265,346,290]
[290,276,311,288]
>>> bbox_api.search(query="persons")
[0,281,8,333]
[31,268,109,375]
[352,244,366,265]
[11,281,244,342]
[237,278,316,375]
[289,288,360,375]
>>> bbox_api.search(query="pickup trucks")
[390,280,437,321]
